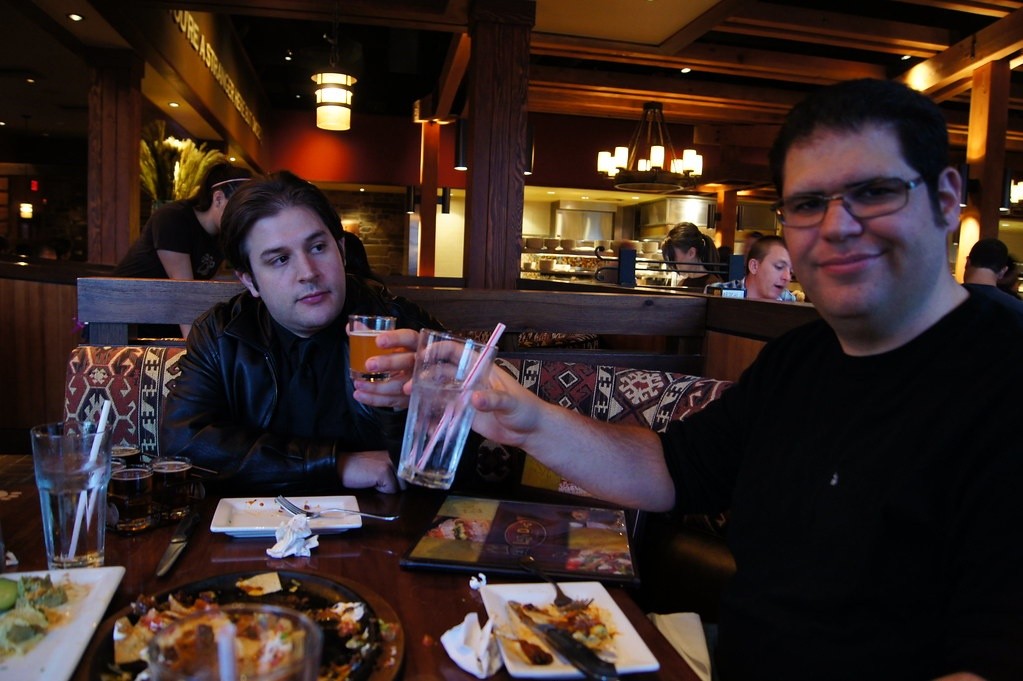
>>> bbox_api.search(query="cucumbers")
[0,577,19,611]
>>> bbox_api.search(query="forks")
[275,495,398,520]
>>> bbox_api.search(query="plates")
[89,568,381,681]
[0,566,126,681]
[209,496,362,537]
[480,582,660,677]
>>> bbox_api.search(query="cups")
[30,421,112,569]
[349,314,396,382]
[102,444,194,536]
[146,604,323,681]
[396,328,498,491]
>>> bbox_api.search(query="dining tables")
[0,484,702,681]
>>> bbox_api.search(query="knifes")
[507,599,619,681]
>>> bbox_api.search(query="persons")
[704,234,796,302]
[401,74,1023,681]
[158,169,485,494]
[959,236,1023,313]
[81,163,255,339]
[661,221,722,287]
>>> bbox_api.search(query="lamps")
[596,103,703,193]
[526,123,535,175]
[311,0,357,130]
[454,119,469,171]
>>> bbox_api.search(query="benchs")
[63,329,736,504]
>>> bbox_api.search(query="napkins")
[646,613,712,681]
[266,513,318,558]
[441,612,503,678]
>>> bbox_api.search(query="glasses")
[770,162,946,230]
